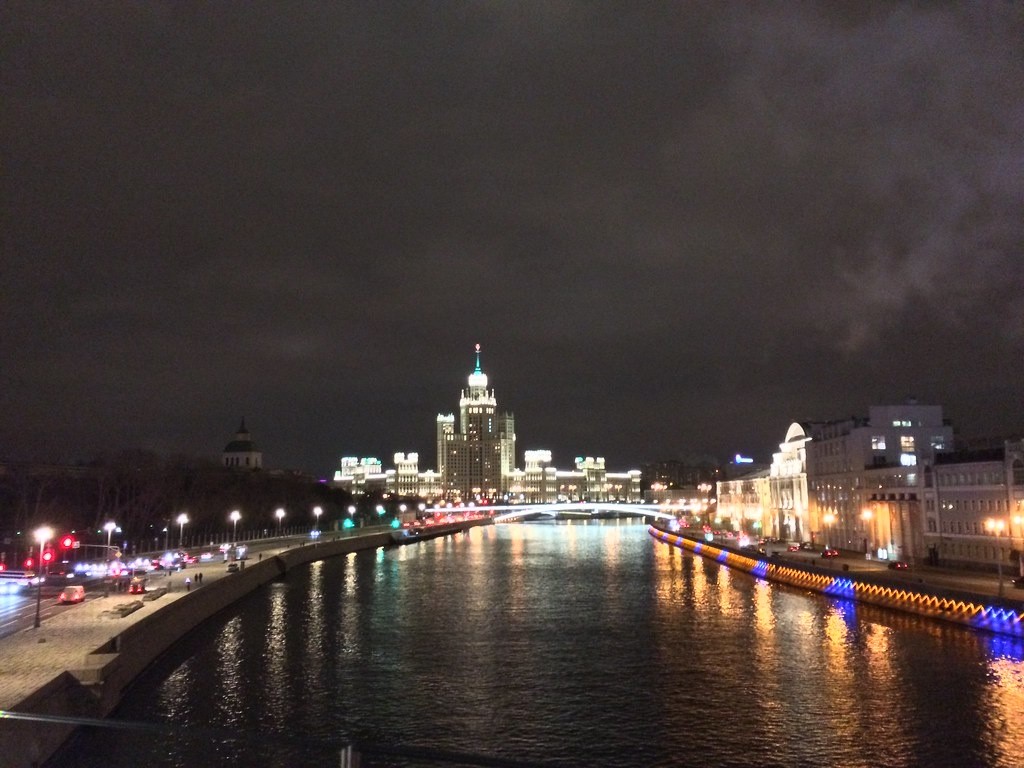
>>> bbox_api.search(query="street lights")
[231,509,240,542]
[375,505,384,529]
[348,505,355,529]
[34,527,52,628]
[177,514,188,550]
[276,508,284,537]
[313,506,322,537]
[400,504,406,522]
[103,520,117,565]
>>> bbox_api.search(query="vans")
[61,585,86,604]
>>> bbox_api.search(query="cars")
[678,518,814,556]
[821,550,838,558]
[18,572,46,591]
[1011,576,1024,588]
[888,561,907,570]
[0,582,20,595]
[311,529,321,535]
[48,544,247,578]
[129,580,145,593]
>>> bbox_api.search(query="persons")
[186,578,191,590]
[259,553,262,561]
[194,573,198,584]
[199,572,203,584]
